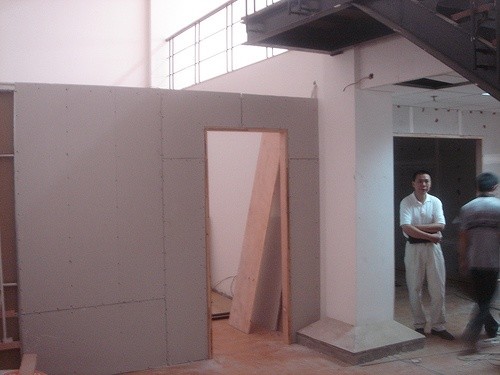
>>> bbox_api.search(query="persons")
[452,173,500,352]
[400,169,455,341]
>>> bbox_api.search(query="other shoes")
[430,328,454,340]
[415,328,426,337]
